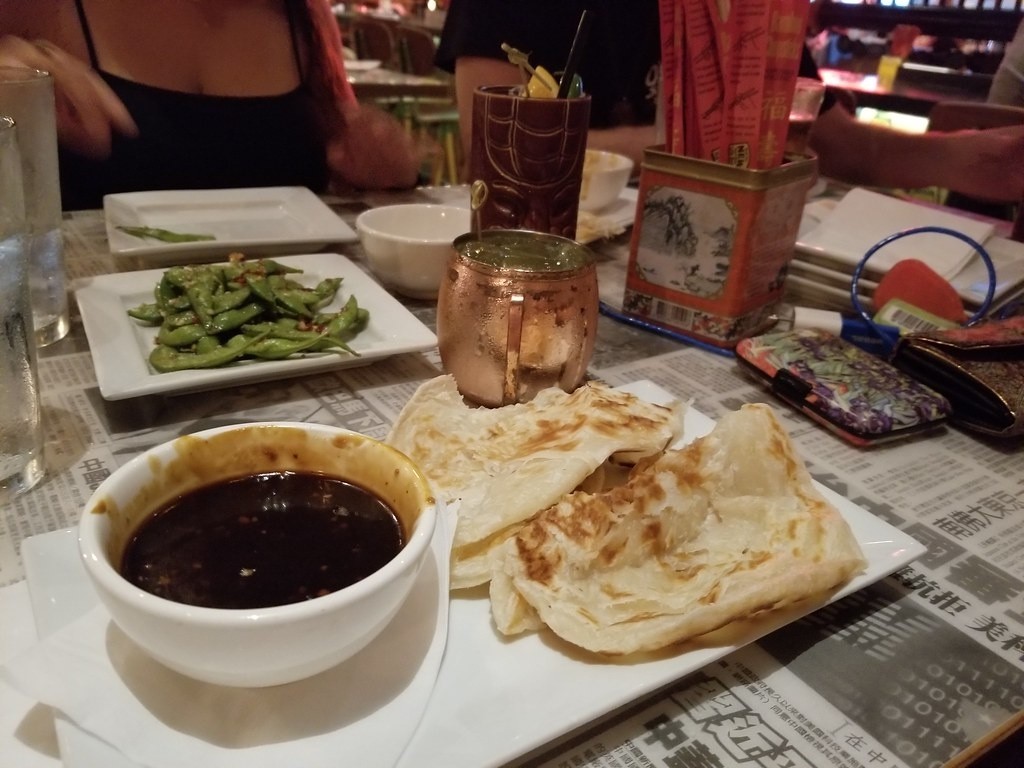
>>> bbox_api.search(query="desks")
[818,68,940,115]
[345,67,451,101]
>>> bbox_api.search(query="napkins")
[10,504,458,768]
[799,188,996,281]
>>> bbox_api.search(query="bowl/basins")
[356,203,472,301]
[580,150,635,213]
[77,420,438,689]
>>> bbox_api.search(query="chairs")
[348,16,413,134]
[394,25,460,186]
[924,99,1024,221]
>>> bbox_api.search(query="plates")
[575,188,639,246]
[21,377,928,768]
[70,253,439,401]
[104,186,358,268]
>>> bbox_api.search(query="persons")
[435,0,1024,201]
[0,1,426,212]
[943,19,1024,220]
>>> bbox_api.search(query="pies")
[488,401,868,657]
[387,371,685,585]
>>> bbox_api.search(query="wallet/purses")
[890,315,1024,438]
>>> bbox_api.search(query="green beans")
[123,252,372,369]
[114,224,216,241]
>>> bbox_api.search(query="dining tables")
[0,178,1024,768]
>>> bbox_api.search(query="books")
[787,186,1023,318]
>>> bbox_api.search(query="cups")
[0,113,47,507]
[0,65,71,350]
[436,229,600,408]
